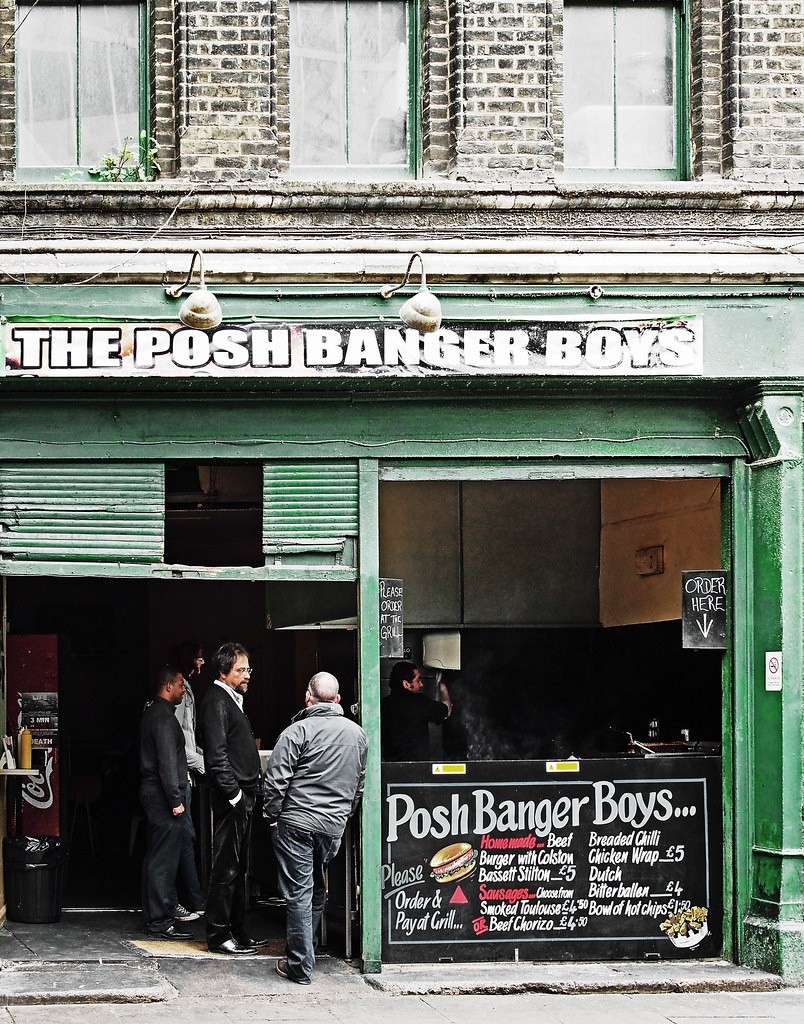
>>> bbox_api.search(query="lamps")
[165,246,222,330]
[379,252,442,334]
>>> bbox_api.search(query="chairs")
[66,775,103,857]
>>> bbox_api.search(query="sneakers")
[174,904,207,921]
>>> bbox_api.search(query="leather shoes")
[235,938,270,948]
[147,923,196,941]
[207,932,258,956]
[276,957,310,985]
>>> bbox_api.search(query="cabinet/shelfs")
[266,474,601,632]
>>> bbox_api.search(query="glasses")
[231,667,254,675]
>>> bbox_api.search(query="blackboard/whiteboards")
[681,570,728,650]
[380,757,724,965]
[378,577,403,658]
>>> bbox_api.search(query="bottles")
[20,726,32,769]
[21,726,24,731]
[18,726,22,769]
[7,737,13,757]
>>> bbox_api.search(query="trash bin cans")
[2,835,62,924]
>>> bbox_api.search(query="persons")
[262,672,369,985]
[198,642,271,956]
[138,640,207,941]
[380,662,453,762]
